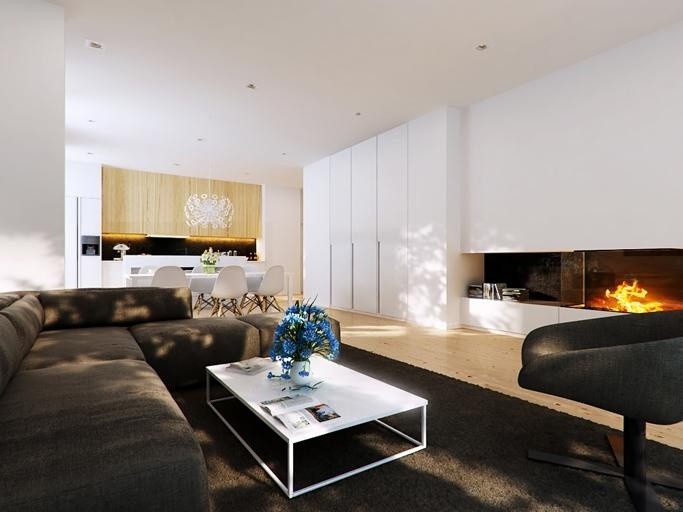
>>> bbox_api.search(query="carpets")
[160,338,681,511]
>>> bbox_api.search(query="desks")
[459,294,582,335]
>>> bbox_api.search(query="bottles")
[216,249,237,257]
[253,251,257,261]
[248,250,252,261]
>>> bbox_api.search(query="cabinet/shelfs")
[351,120,408,323]
[189,177,263,239]
[101,165,190,236]
[300,146,353,314]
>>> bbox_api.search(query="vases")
[289,359,314,384]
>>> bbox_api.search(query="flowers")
[199,246,220,264]
[266,293,343,379]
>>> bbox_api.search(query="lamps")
[181,143,237,230]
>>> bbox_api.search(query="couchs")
[0,287,342,511]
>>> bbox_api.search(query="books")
[257,391,343,431]
[468,283,529,302]
[224,356,275,377]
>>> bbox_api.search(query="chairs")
[125,262,293,317]
[516,308,682,511]
